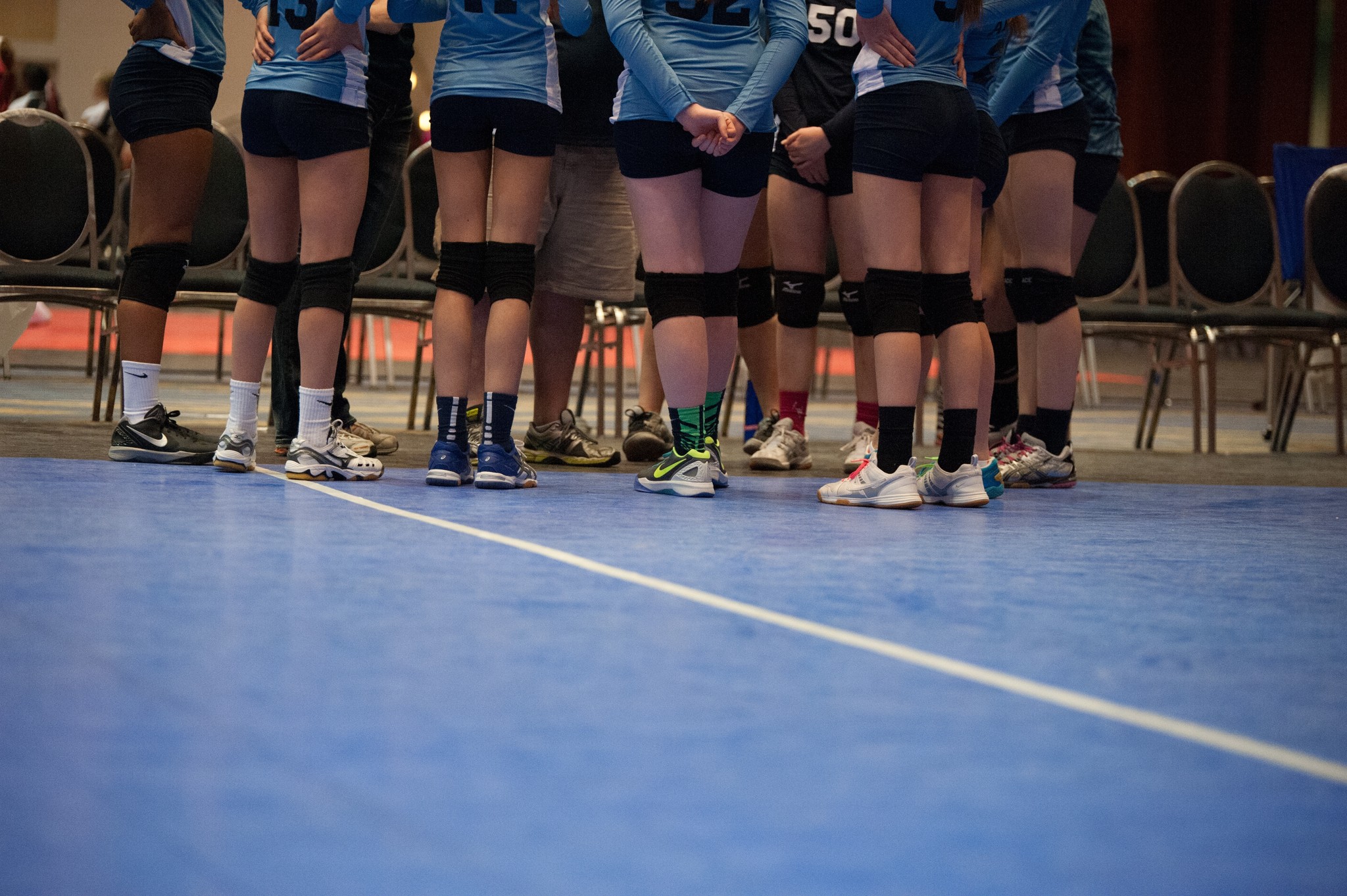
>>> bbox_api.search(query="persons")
[0,0,1124,510]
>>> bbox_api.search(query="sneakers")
[463,404,484,465]
[213,428,258,472]
[995,433,1077,488]
[274,426,376,458]
[345,420,399,454]
[521,408,622,466]
[426,440,475,487]
[634,446,715,498]
[284,419,385,481]
[622,405,674,461]
[816,451,922,508]
[474,437,538,488]
[990,427,1024,461]
[981,457,1005,500]
[704,437,729,487]
[748,417,813,471]
[838,420,875,473]
[742,409,782,457]
[915,454,989,507]
[108,402,220,463]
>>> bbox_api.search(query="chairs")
[0,109,1347,457]
